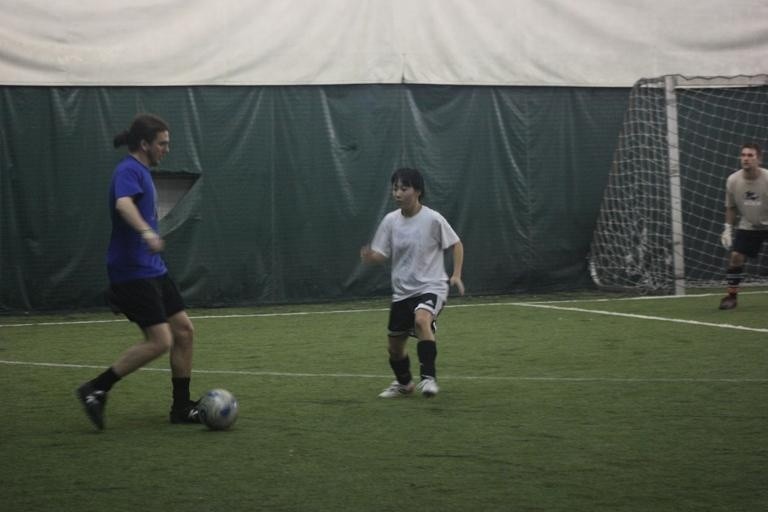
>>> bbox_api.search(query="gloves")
[721,223,734,249]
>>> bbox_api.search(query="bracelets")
[140,228,154,236]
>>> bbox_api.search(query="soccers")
[198,387,238,429]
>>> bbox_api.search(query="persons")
[360,168,466,400]
[718,142,768,309]
[73,113,204,433]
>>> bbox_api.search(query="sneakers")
[378,379,415,398]
[171,400,202,423]
[75,381,107,432]
[422,379,439,395]
[720,296,738,309]
